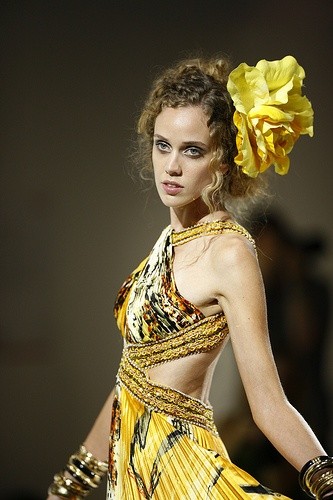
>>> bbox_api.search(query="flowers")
[226,55,315,178]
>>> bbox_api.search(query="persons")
[44,52,333,500]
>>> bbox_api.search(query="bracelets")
[47,443,111,500]
[299,453,333,500]
